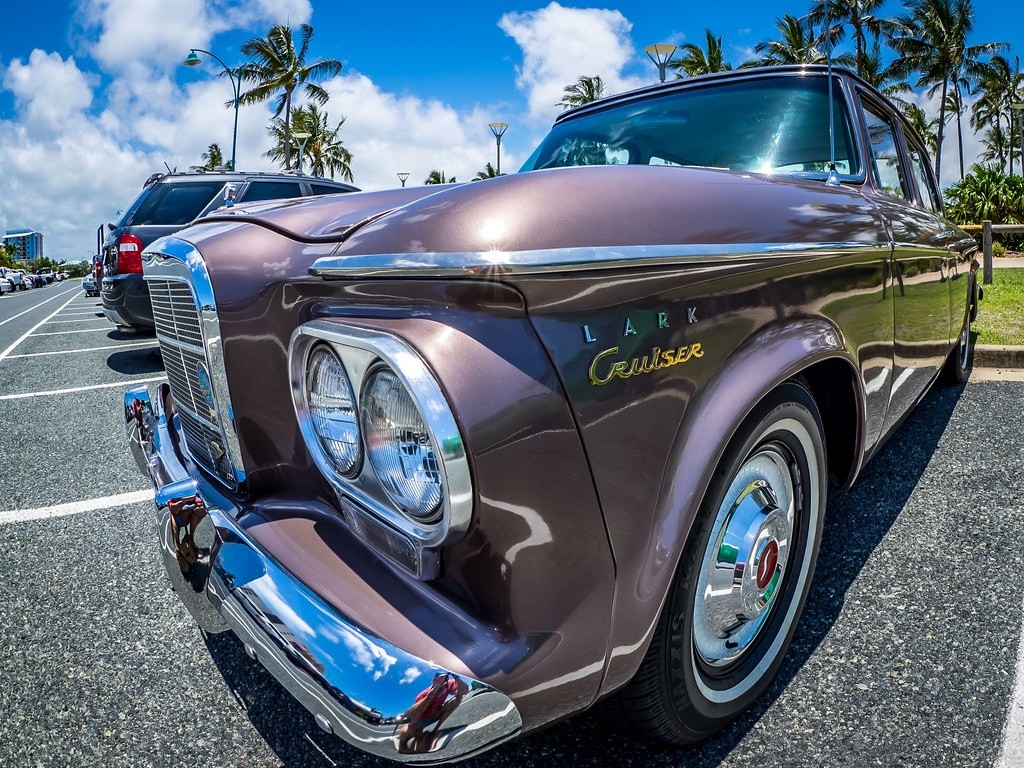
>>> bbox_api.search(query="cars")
[123,66,985,767]
[0,266,72,292]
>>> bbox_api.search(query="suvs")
[100,173,362,337]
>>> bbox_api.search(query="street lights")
[291,129,313,170]
[396,170,412,192]
[488,120,510,170]
[646,42,677,80]
[185,53,243,170]
